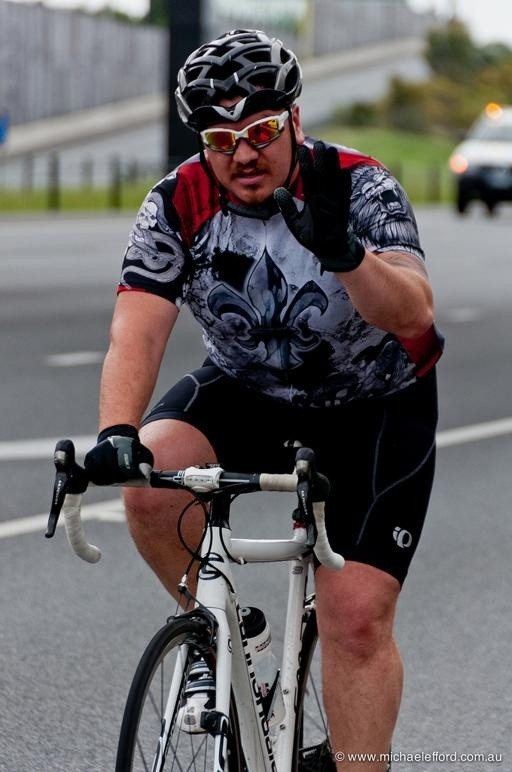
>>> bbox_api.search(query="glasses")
[198,113,288,155]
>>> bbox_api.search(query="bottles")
[237,607,286,730]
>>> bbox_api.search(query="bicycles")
[42,438,336,770]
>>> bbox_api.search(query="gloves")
[82,425,154,485]
[271,138,365,272]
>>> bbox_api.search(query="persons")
[78,22,443,772]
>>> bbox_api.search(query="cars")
[450,107,512,213]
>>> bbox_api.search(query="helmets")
[173,28,302,134]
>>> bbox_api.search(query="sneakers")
[173,644,217,733]
